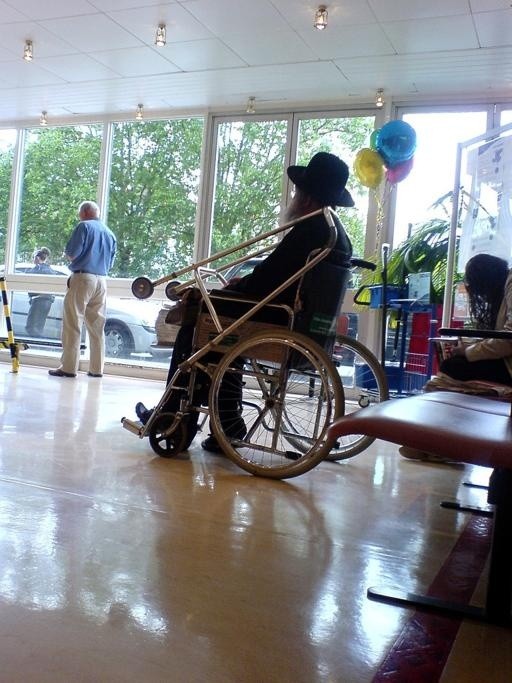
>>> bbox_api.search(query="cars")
[0,262,173,359]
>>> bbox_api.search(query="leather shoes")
[48,369,76,377]
[88,371,103,377]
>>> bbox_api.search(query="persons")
[25,246,55,338]
[137,152,356,449]
[49,197,117,377]
[399,253,512,462]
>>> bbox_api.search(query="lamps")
[314,4,327,29]
[245,95,256,113]
[134,103,144,120]
[23,39,33,60]
[375,87,384,106]
[38,110,47,126]
[154,23,166,45]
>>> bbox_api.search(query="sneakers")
[399,446,464,462]
[204,417,247,451]
[141,408,198,451]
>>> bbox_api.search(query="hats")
[286,152,355,208]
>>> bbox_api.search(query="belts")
[74,270,104,276]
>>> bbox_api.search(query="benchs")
[323,327,512,623]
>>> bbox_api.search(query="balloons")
[352,118,417,193]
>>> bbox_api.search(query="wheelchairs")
[120,248,391,481]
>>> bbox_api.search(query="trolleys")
[352,243,438,408]
